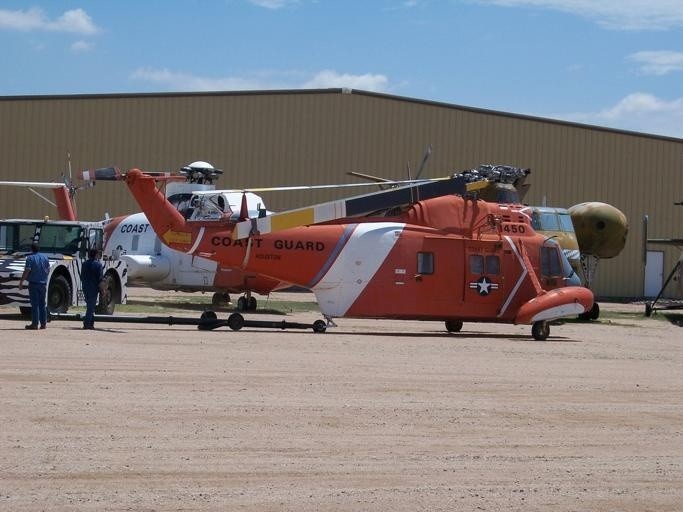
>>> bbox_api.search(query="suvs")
[0,220,128,313]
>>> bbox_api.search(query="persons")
[19,243,51,329]
[80,248,106,330]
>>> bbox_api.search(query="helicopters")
[0,159,277,310]
[75,146,594,342]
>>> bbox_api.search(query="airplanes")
[641,202,683,318]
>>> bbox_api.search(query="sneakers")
[25,322,38,330]
[39,324,46,329]
